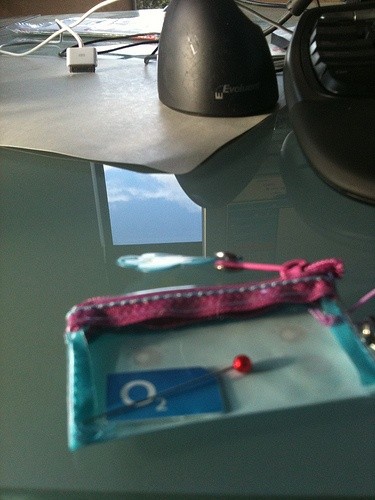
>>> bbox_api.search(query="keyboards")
[284,1,375,205]
